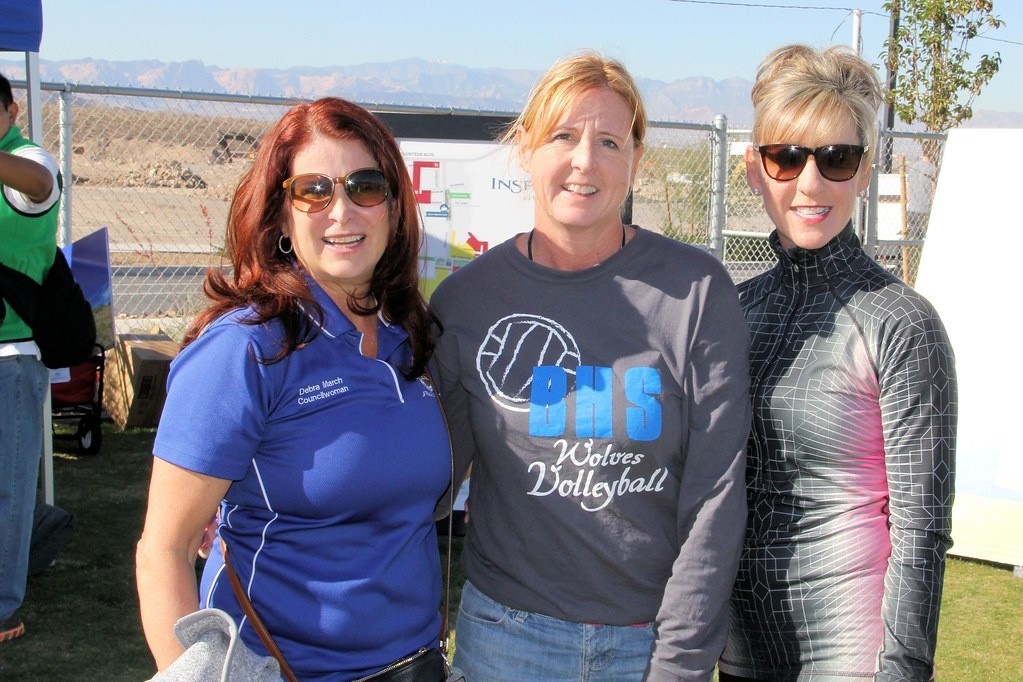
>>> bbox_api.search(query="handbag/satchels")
[349,647,451,682]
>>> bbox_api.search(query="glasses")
[752,143,868,182]
[281,167,389,213]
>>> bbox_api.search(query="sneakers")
[0,612,25,644]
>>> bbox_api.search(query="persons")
[1,73,103,642]
[418,48,754,682]
[136,98,453,682]
[718,42,958,681]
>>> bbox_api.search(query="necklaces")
[528,223,626,274]
